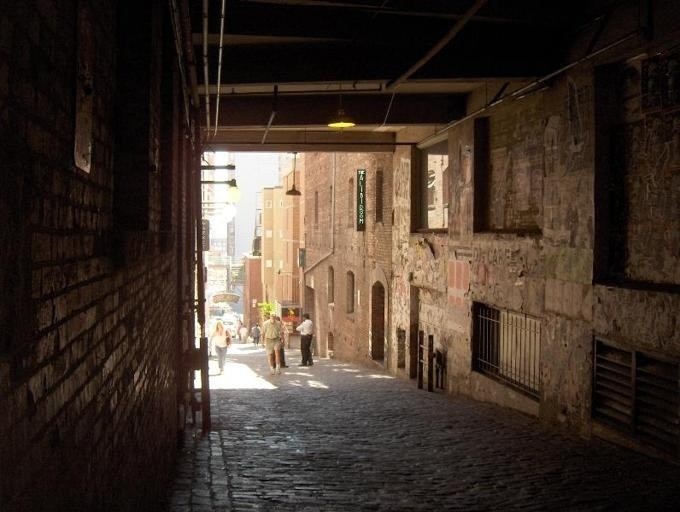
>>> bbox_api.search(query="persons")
[237,312,290,374]
[209,320,232,372]
[295,313,314,366]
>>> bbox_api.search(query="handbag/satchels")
[227,338,231,346]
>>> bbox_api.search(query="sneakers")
[271,369,282,375]
[281,365,289,368]
[299,363,314,366]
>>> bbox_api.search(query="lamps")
[327,89,357,128]
[283,152,303,197]
